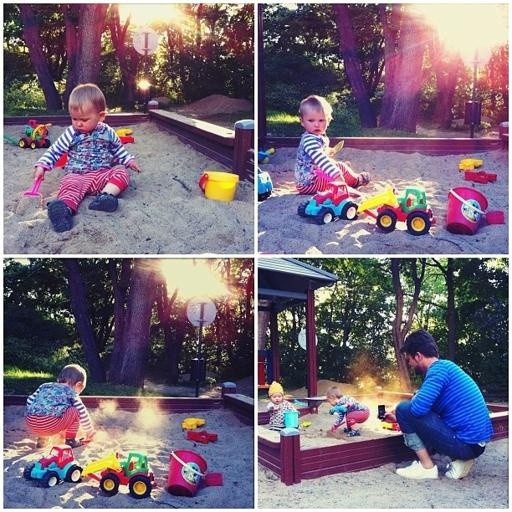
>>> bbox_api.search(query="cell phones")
[378,405,385,419]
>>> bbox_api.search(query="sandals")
[347,429,360,437]
[64,439,81,448]
[49,200,70,233]
[88,194,118,211]
[352,171,370,188]
[343,427,351,433]
[36,437,52,447]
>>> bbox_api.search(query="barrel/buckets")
[446,186,488,235]
[167,449,207,497]
[198,171,239,201]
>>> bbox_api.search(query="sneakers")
[445,457,473,479]
[396,462,438,479]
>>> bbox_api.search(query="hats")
[269,381,283,397]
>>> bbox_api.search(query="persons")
[326,386,369,437]
[293,95,372,194]
[17,120,51,149]
[378,329,494,481]
[25,364,96,449]
[266,381,301,432]
[33,84,142,233]
